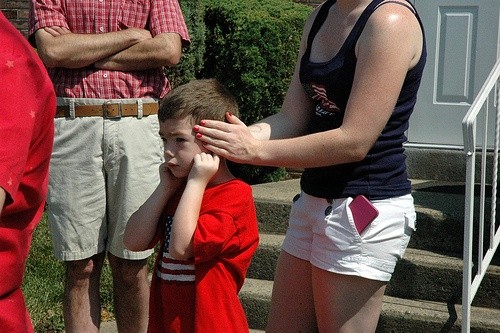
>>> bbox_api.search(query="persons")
[123,78,260,333]
[193,0,427,333]
[28,0,191,333]
[0,10,57,333]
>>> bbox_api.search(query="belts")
[54,101,159,119]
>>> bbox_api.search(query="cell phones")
[348,194,379,234]
